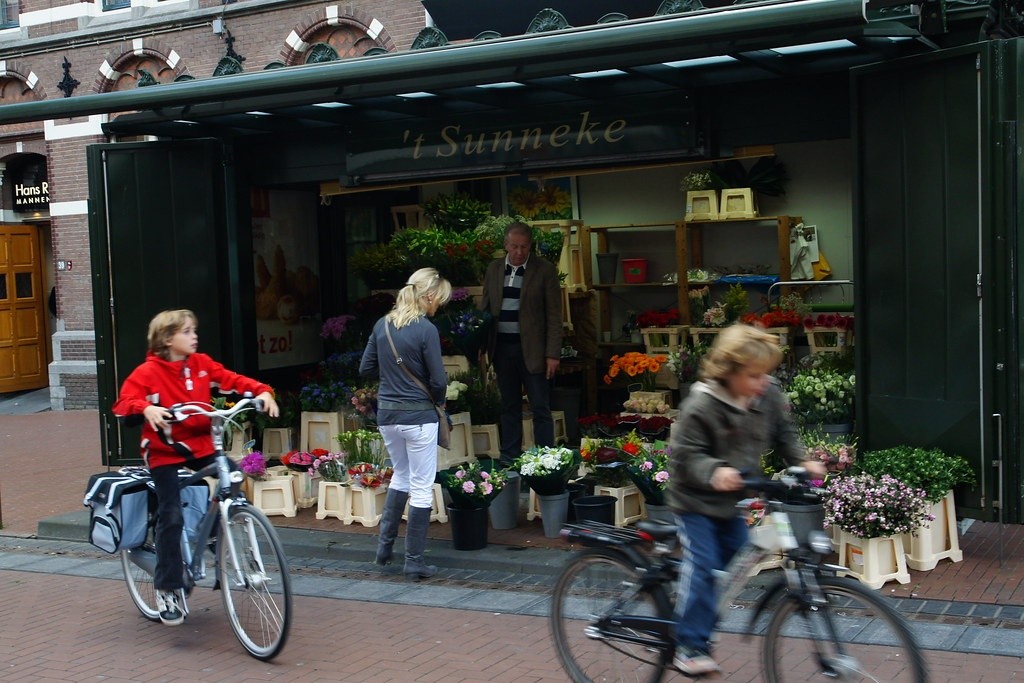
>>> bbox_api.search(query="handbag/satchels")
[83,471,150,554]
[178,469,210,541]
[432,403,452,452]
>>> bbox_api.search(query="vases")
[595,253,620,284]
[781,503,826,543]
[804,423,851,463]
[629,391,673,408]
[538,489,571,538]
[631,329,642,344]
[571,495,618,526]
[447,504,489,551]
[488,471,520,529]
[644,501,678,550]
[623,258,648,283]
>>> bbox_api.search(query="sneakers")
[155,589,186,626]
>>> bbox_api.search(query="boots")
[403,504,437,581]
[375,487,409,566]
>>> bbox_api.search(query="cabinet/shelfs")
[574,214,803,430]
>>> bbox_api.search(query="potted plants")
[346,192,588,293]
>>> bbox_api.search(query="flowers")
[210,287,501,427]
[509,444,584,495]
[279,428,395,487]
[445,240,496,266]
[579,284,977,539]
[439,457,509,509]
[680,170,712,192]
[240,451,268,481]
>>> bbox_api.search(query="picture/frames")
[500,176,580,225]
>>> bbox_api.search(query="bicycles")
[547,468,930,683]
[85,394,295,661]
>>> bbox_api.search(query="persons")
[112,310,280,626]
[668,325,826,674]
[359,267,454,583]
[481,223,563,468]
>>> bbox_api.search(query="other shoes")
[671,649,720,674]
